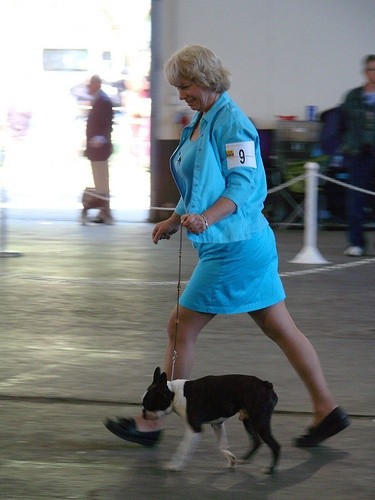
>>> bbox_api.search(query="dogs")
[81,189,114,225]
[141,366,283,474]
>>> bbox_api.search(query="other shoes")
[343,246,365,256]
[89,213,111,223]
[294,405,350,448]
[103,414,163,447]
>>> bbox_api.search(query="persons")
[118,72,152,160]
[317,54,375,258]
[83,75,114,219]
[100,46,351,450]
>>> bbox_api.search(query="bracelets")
[202,215,208,229]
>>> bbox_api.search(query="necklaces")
[177,126,200,163]
